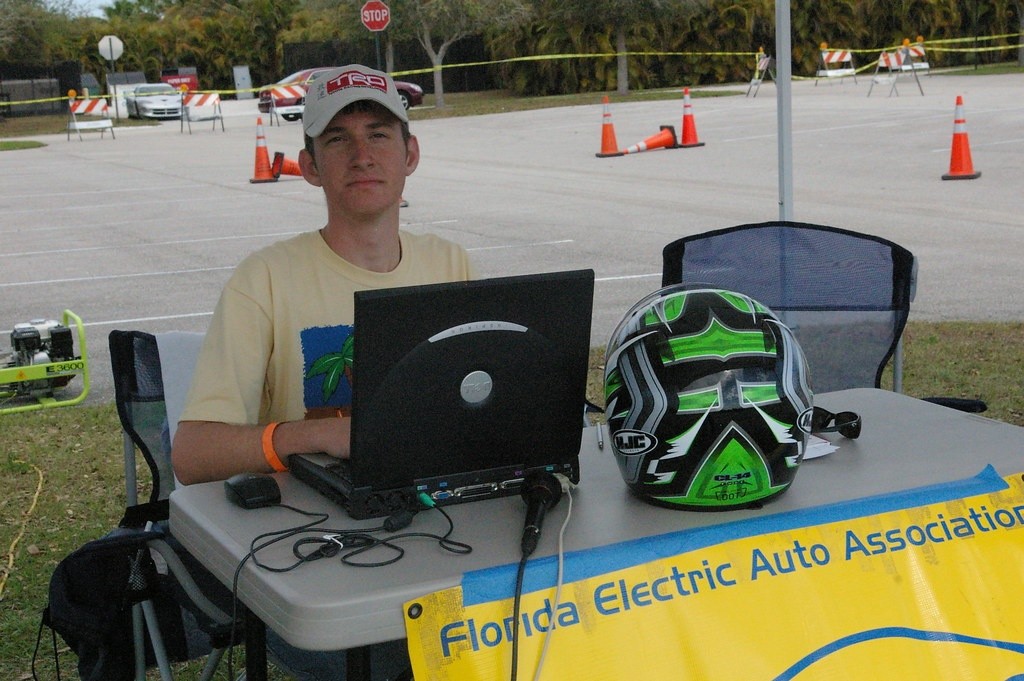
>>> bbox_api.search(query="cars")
[258,67,425,122]
[126,83,187,120]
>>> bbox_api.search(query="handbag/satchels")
[32,532,244,681]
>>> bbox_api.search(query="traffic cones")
[271,150,304,177]
[622,125,680,154]
[677,87,707,147]
[248,116,280,184]
[595,96,626,158]
[942,96,983,180]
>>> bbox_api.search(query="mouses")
[225,472,282,509]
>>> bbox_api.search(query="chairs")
[37,328,245,681]
[662,221,990,415]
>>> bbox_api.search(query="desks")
[169,389,1024,680]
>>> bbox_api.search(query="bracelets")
[262,421,287,473]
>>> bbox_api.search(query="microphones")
[518,470,562,555]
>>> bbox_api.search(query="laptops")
[288,268,596,521]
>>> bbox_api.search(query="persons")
[171,65,474,681]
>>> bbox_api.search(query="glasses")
[811,407,861,438]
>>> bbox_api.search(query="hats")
[302,65,408,142]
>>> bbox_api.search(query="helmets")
[606,281,814,513]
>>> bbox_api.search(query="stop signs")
[360,0,392,32]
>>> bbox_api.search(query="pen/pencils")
[596,421,603,450]
